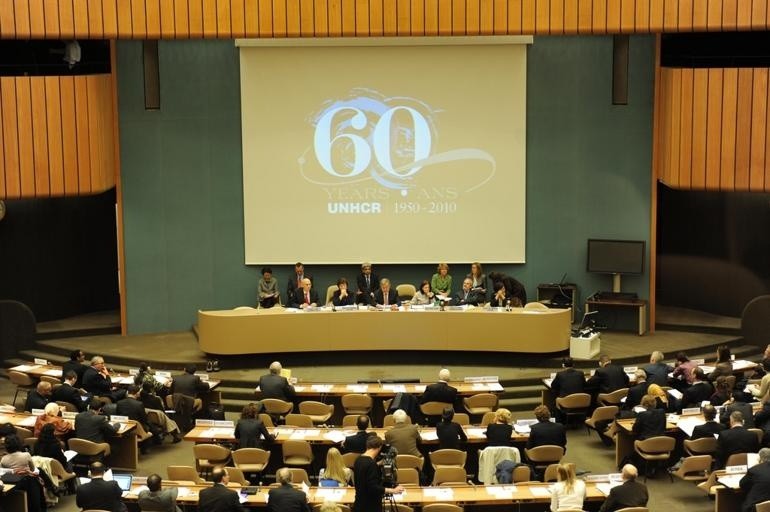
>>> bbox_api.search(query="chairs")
[429,448,467,470]
[33,455,76,487]
[55,401,79,412]
[340,393,373,415]
[396,469,419,486]
[512,466,530,482]
[129,420,153,442]
[747,428,764,443]
[422,503,464,512]
[40,376,62,386]
[481,412,496,425]
[14,425,33,445]
[478,447,520,470]
[421,402,453,416]
[683,438,717,456]
[462,392,497,415]
[396,454,419,469]
[191,444,232,481]
[555,393,591,427]
[285,414,313,427]
[725,453,748,466]
[258,414,275,427]
[524,446,564,462]
[597,388,630,407]
[585,406,619,429]
[276,468,310,485]
[68,438,110,468]
[24,438,39,451]
[661,386,673,394]
[382,504,414,512]
[258,399,294,420]
[697,470,726,493]
[383,399,393,412]
[433,468,467,486]
[298,402,335,422]
[755,500,770,512]
[282,440,315,482]
[225,467,246,485]
[634,436,676,483]
[9,371,34,410]
[166,465,200,481]
[342,452,362,468]
[165,395,202,411]
[230,448,271,481]
[342,415,372,429]
[452,413,469,425]
[325,285,339,306]
[544,464,560,482]
[383,415,412,428]
[396,284,417,299]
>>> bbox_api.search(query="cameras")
[375,444,399,489]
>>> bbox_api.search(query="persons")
[738,448,770,505]
[586,354,629,408]
[343,415,377,452]
[287,279,320,308]
[678,367,714,414]
[139,476,182,512]
[673,351,699,387]
[51,369,83,406]
[383,412,422,461]
[0,436,68,512]
[117,387,152,432]
[137,381,181,444]
[172,363,211,407]
[199,469,241,512]
[746,357,770,406]
[713,411,760,471]
[488,284,511,307]
[79,357,111,400]
[357,263,379,305]
[640,351,674,388]
[437,409,468,452]
[527,406,566,454]
[623,370,657,415]
[431,263,452,305]
[409,281,437,305]
[691,404,725,441]
[62,350,90,386]
[75,399,126,465]
[352,436,405,511]
[708,347,733,382]
[266,469,311,512]
[328,279,355,305]
[550,463,587,512]
[422,370,457,407]
[258,268,280,310]
[633,396,666,443]
[76,461,126,512]
[259,362,296,405]
[469,263,496,306]
[22,382,52,412]
[484,408,514,447]
[235,403,279,448]
[549,356,590,421]
[0,424,20,449]
[456,278,475,305]
[135,364,166,397]
[371,279,399,308]
[292,262,312,290]
[324,430,353,484]
[35,403,74,438]
[720,390,753,428]
[32,424,76,475]
[600,464,648,512]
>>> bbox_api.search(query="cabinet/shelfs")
[569,332,601,360]
[537,285,575,323]
[585,297,647,336]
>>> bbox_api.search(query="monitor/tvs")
[587,238,646,276]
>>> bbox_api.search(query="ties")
[366,274,370,288]
[299,275,301,280]
[304,291,309,304]
[384,291,388,305]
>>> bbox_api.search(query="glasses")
[95,362,104,366]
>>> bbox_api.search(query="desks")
[0,413,136,449]
[8,362,63,387]
[194,302,575,361]
[670,455,712,481]
[106,474,629,509]
[542,359,755,390]
[718,474,746,493]
[255,381,502,404]
[616,406,766,437]
[183,425,536,462]
[109,372,219,393]
